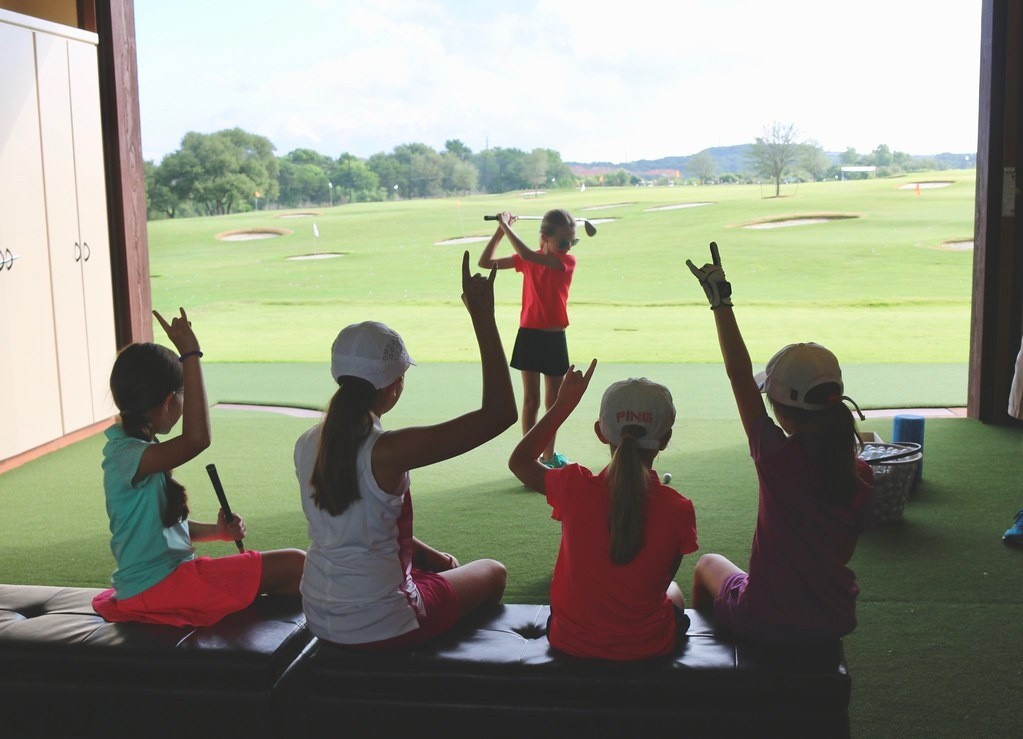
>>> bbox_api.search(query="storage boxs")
[852,430,883,444]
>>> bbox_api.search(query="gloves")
[686,241,734,310]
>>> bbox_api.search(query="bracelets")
[179,351,203,362]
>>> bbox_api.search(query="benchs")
[0,582,315,739]
[286,601,852,739]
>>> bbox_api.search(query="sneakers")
[1001,509,1023,542]
[540,453,569,469]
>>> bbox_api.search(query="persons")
[1002,333,1023,544]
[687,242,873,645]
[477,208,581,469]
[92,306,306,629]
[293,250,518,650]
[509,358,699,660]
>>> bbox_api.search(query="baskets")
[854,442,922,523]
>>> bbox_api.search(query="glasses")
[545,238,580,249]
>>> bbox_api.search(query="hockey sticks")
[205,463,245,555]
[483,214,597,238]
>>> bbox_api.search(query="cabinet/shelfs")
[0,8,121,475]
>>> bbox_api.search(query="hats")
[599,377,677,450]
[331,321,416,390]
[754,342,844,411]
